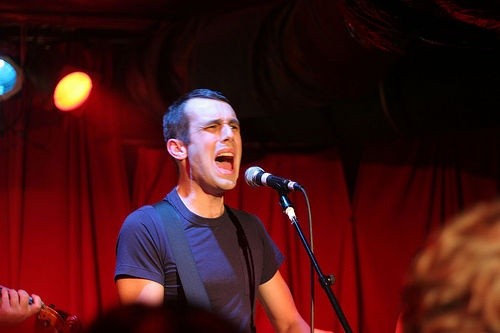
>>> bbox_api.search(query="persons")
[114,88,334,333]
[395,200,499,333]
[0,284,43,333]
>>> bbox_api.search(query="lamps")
[24,42,93,111]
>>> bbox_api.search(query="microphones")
[244,166,302,192]
